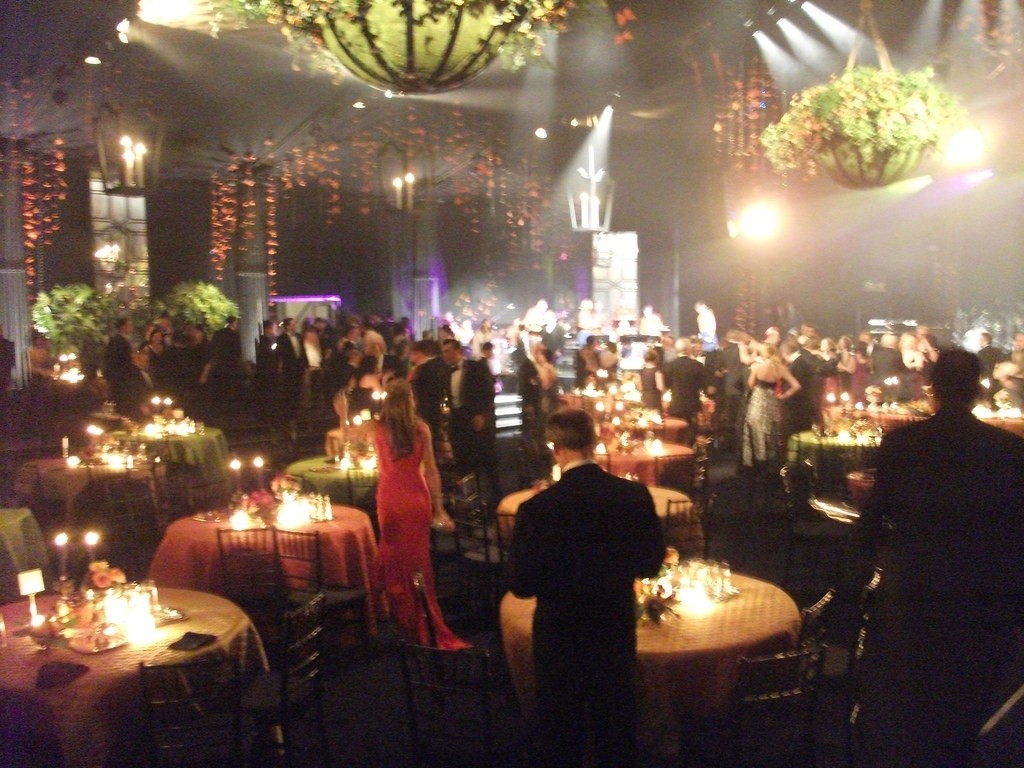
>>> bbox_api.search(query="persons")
[508,411,670,768]
[0,295,1024,528]
[843,348,1024,767]
[330,377,476,653]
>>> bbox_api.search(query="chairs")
[0,417,1024,768]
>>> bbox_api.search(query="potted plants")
[199,0,608,95]
[759,1,970,190]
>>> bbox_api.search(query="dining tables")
[28,454,166,522]
[325,427,372,457]
[143,498,381,660]
[0,583,285,768]
[108,428,233,504]
[788,428,883,496]
[595,442,698,486]
[284,454,424,508]
[496,484,705,559]
[600,417,688,446]
[498,560,800,760]
[0,507,51,593]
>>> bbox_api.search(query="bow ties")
[450,366,460,373]
